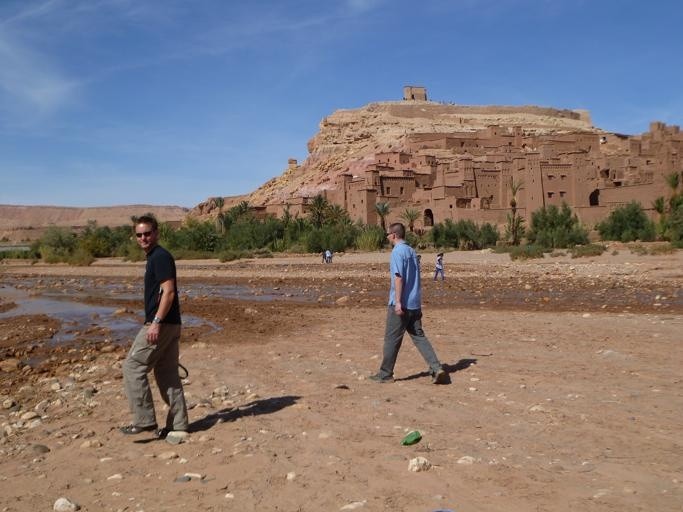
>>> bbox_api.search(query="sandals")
[119,421,159,435]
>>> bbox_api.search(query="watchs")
[153,317,163,324]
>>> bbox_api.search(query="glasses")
[135,231,154,237]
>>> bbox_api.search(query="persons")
[368,223,446,384]
[121,217,189,436]
[433,253,446,282]
[322,248,332,263]
[417,255,421,263]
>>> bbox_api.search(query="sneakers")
[431,369,446,384]
[365,373,397,383]
